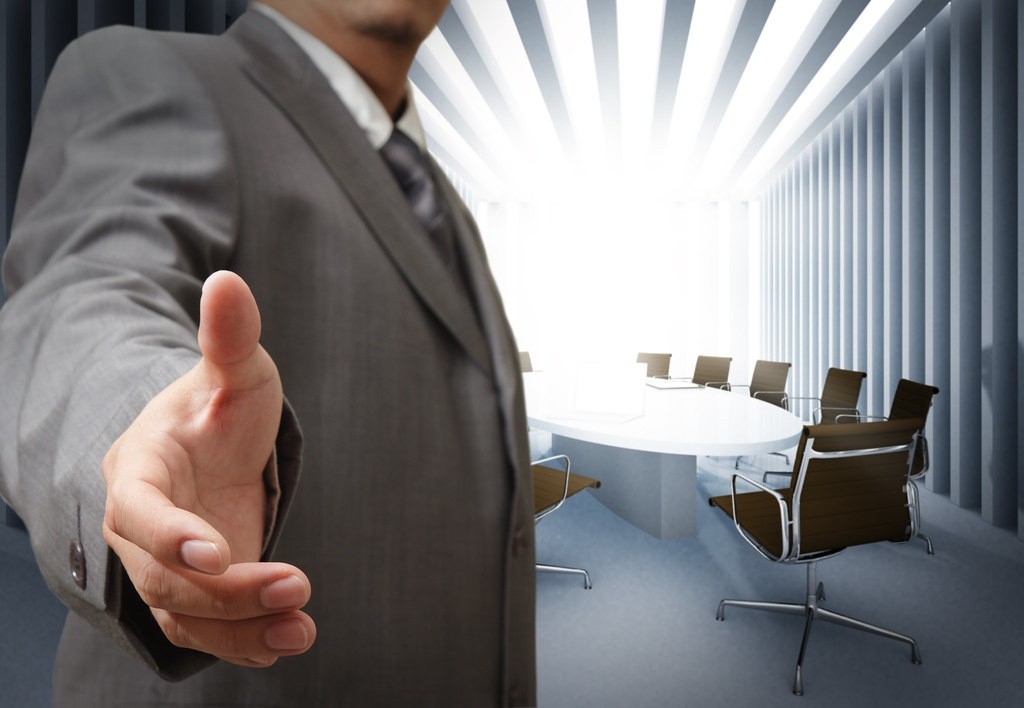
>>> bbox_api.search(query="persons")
[0,0,538,708]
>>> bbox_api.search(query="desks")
[522,368,805,543]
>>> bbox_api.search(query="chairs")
[532,453,601,590]
[763,367,867,483]
[708,416,925,697]
[520,351,533,372]
[671,355,732,392]
[835,378,940,555]
[706,360,792,470]
[637,353,673,380]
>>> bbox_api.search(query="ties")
[376,125,465,289]
